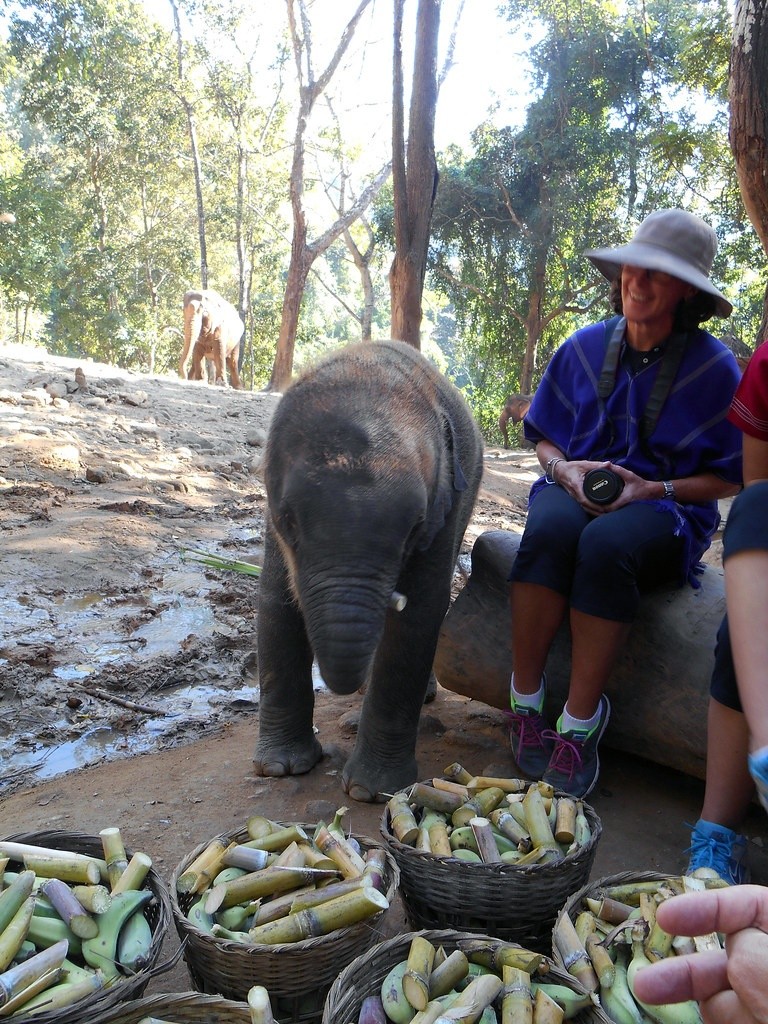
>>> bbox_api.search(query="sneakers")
[509,670,551,778]
[748,746,768,810]
[682,817,750,890]
[544,693,611,800]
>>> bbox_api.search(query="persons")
[682,330,768,886]
[633,884,768,1024]
[502,208,745,800]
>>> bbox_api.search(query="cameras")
[583,468,624,502]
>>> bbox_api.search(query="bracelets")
[544,458,565,485]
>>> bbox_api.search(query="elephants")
[499,393,537,452]
[177,289,246,390]
[249,338,486,808]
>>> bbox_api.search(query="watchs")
[661,481,676,501]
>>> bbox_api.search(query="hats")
[584,209,732,320]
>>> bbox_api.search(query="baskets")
[83,991,280,1024]
[380,776,603,951]
[321,929,611,1024]
[171,819,400,1024]
[0,828,174,1024]
[550,870,735,1024]
